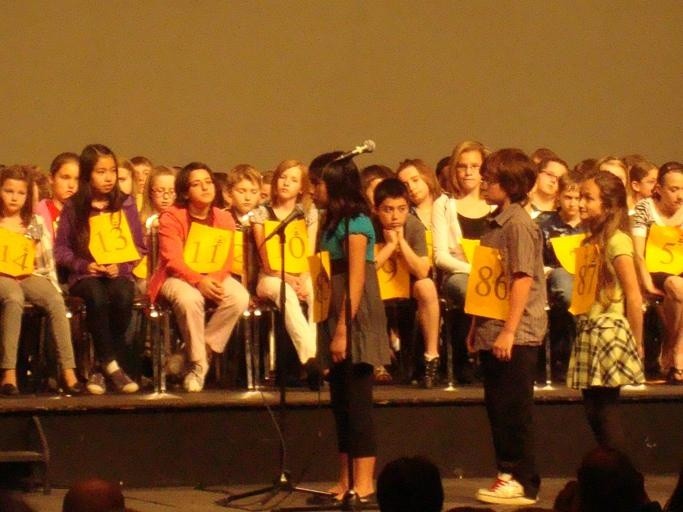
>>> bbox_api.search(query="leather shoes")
[0,383,20,398]
[66,382,85,393]
[305,490,378,510]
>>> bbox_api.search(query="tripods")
[270,166,378,512]
[215,232,338,507]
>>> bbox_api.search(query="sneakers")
[373,369,393,384]
[476,470,540,505]
[168,354,184,374]
[423,352,441,389]
[644,368,670,385]
[85,372,106,395]
[108,367,140,394]
[183,364,209,393]
[308,370,324,391]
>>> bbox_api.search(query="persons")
[305,152,394,512]
[548,480,586,511]
[461,147,551,507]
[55,475,133,512]
[577,445,661,511]
[367,455,445,511]
[0,139,339,396]
[564,168,652,462]
[359,141,681,394]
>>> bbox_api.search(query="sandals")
[666,366,683,385]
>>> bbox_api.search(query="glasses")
[541,169,560,182]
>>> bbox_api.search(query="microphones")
[334,140,375,161]
[266,204,304,240]
